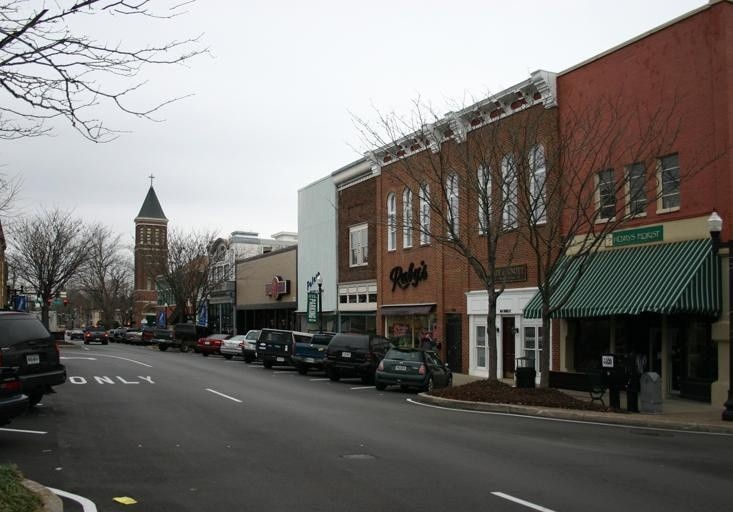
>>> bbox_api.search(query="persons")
[419,328,433,347]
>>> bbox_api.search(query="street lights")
[707,208,733,421]
[317,274,324,334]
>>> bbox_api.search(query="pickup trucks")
[150,322,217,353]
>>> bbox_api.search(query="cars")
[82,325,109,345]
[70,327,85,341]
[0,311,66,421]
[219,334,246,359]
[196,333,234,356]
[374,344,454,395]
[106,326,165,345]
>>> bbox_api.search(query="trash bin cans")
[640,372,663,413]
[515,357,536,387]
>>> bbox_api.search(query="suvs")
[241,329,261,363]
[255,328,315,369]
[323,328,393,386]
[295,331,337,377]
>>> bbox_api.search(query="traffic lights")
[63,299,68,307]
[48,297,52,306]
